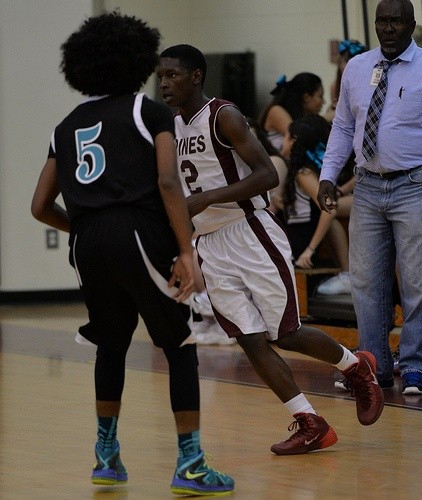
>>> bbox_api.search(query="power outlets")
[46,229,58,248]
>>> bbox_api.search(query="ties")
[361,60,399,162]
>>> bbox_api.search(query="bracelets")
[335,187,344,196]
[306,245,316,254]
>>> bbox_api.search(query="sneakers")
[170,450,235,496]
[340,351,385,426]
[90,439,128,487]
[270,412,338,455]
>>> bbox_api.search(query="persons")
[32,11,235,496]
[258,40,370,295]
[316,0,422,394]
[155,44,385,455]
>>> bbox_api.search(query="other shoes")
[317,271,352,294]
[401,372,422,394]
[391,345,400,373]
[334,369,395,390]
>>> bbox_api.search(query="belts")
[369,167,421,178]
